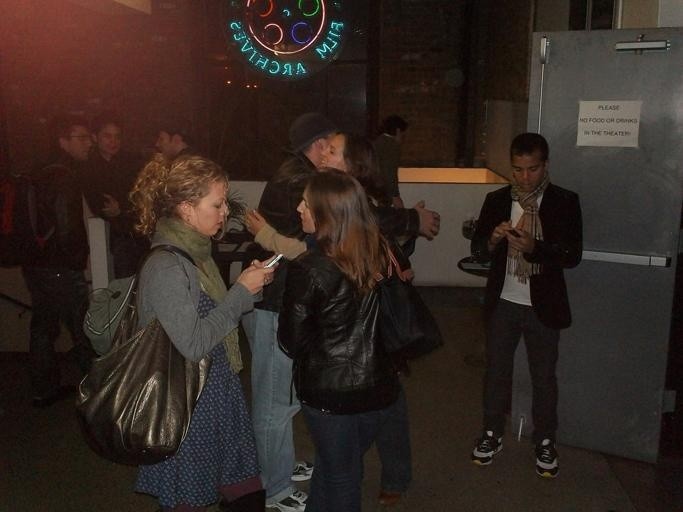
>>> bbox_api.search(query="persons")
[128,153,278,512]
[9,111,196,404]
[242,116,439,512]
[470,132,583,480]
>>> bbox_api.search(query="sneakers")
[534,438,561,480]
[265,488,312,512]
[33,386,77,408]
[471,431,503,466]
[290,461,314,482]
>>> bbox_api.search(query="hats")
[289,112,342,155]
[377,112,409,135]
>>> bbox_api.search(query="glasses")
[69,133,98,143]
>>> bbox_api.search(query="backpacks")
[79,245,197,356]
[0,163,80,267]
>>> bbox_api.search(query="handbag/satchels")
[74,250,213,466]
[359,232,444,375]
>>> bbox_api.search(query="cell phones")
[508,227,521,236]
[263,254,283,268]
